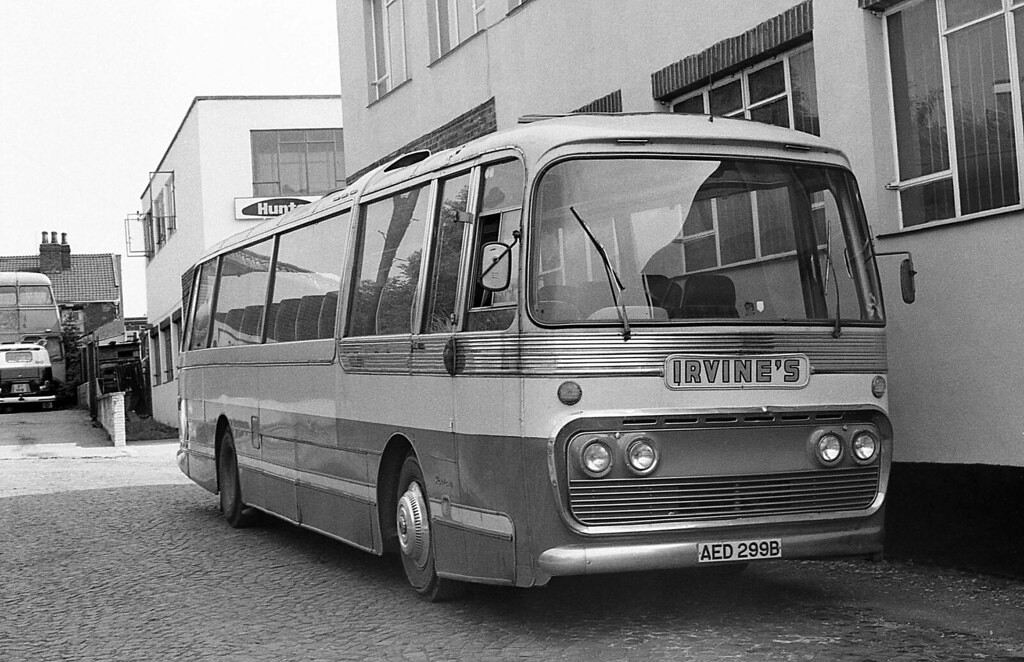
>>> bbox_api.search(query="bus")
[0,271,68,397]
[175,113,921,604]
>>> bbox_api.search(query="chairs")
[681,272,741,319]
[257,303,280,339]
[240,305,263,336]
[294,295,324,341]
[535,299,582,324]
[274,298,301,342]
[215,312,227,323]
[626,273,683,318]
[374,276,401,333]
[316,290,338,339]
[539,283,603,320]
[578,280,620,309]
[225,308,244,332]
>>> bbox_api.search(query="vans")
[0,344,57,406]
